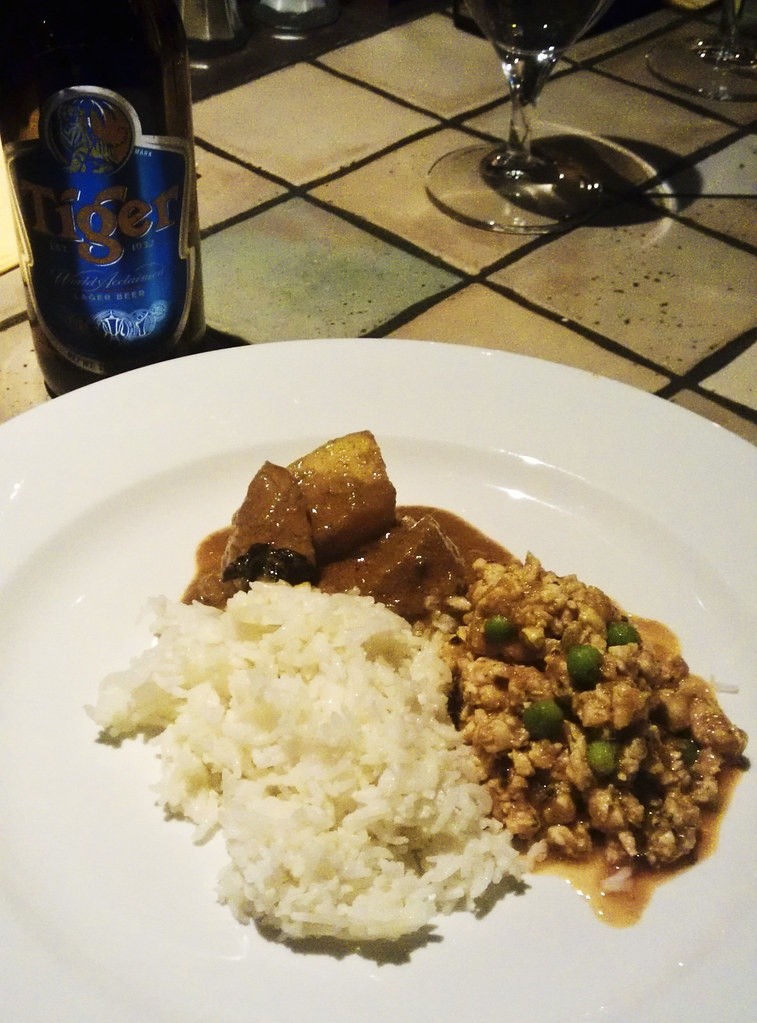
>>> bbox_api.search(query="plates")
[0,339,757,1023]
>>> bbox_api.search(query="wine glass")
[423,0,605,234]
[644,0,757,101]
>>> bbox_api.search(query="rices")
[81,577,633,947]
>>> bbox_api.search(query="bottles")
[0,0,204,394]
[180,0,247,58]
[254,0,341,30]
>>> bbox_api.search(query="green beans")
[484,610,641,780]
